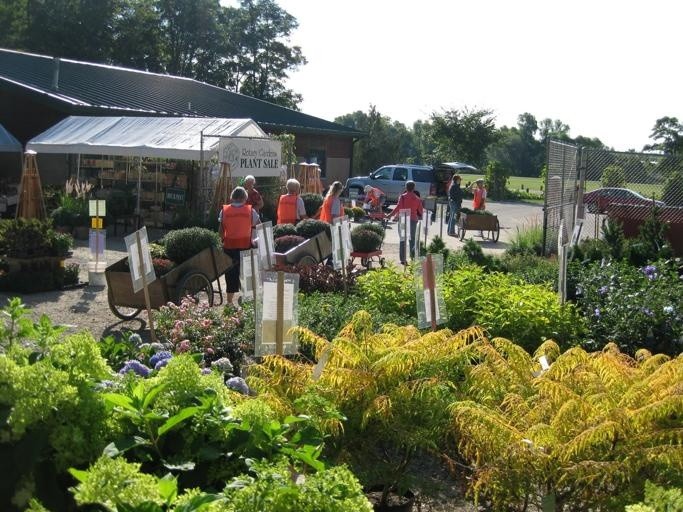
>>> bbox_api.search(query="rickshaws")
[104,238,238,320]
[457,208,500,243]
[274,227,332,274]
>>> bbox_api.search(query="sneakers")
[447,230,483,237]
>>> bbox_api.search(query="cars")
[581,187,665,214]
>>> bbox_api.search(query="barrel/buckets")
[87,262,107,289]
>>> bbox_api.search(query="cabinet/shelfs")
[68,154,165,237]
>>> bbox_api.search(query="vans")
[343,161,478,206]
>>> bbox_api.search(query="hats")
[363,184,373,191]
[452,173,484,184]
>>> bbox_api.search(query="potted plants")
[48,191,88,240]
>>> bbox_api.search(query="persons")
[360,185,388,214]
[464,177,485,238]
[446,174,464,236]
[274,178,309,227]
[213,186,263,307]
[316,180,344,267]
[383,180,423,265]
[236,174,264,212]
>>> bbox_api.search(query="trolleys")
[349,219,388,267]
